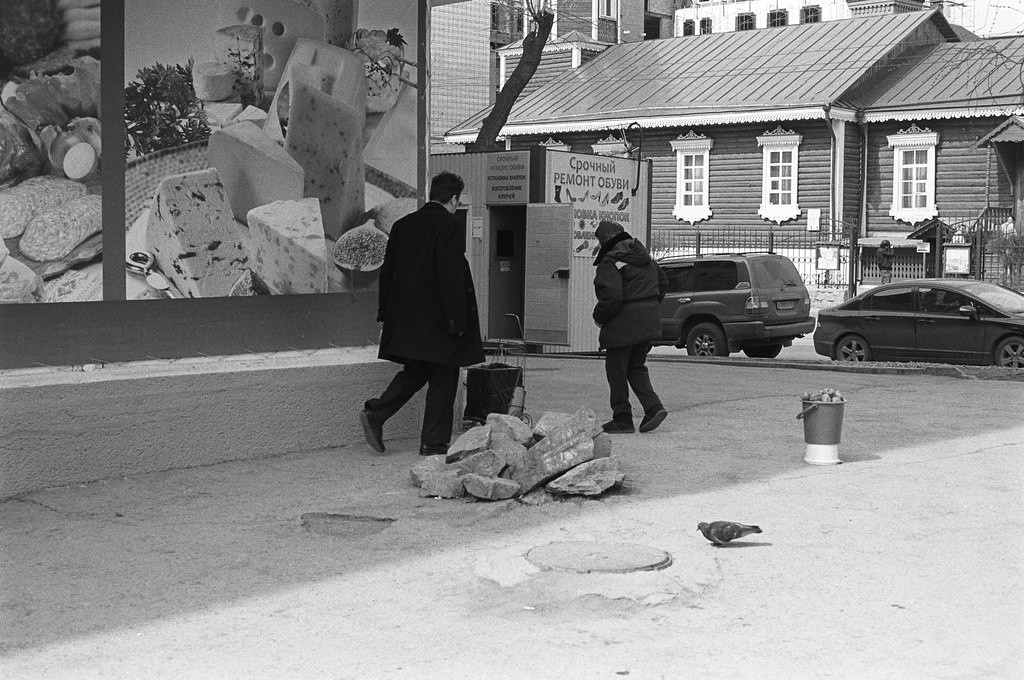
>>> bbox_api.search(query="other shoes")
[359,408,386,453]
[602,418,636,434]
[420,442,450,456]
[639,404,668,433]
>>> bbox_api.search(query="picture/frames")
[493,228,519,260]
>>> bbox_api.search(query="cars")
[813,278,1024,368]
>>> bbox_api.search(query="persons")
[1001,215,1017,240]
[592,221,669,433]
[877,240,894,284]
[360,171,486,456]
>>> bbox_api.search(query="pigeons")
[697,521,762,544]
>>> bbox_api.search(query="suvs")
[647,251,816,356]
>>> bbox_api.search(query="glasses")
[458,198,462,207]
